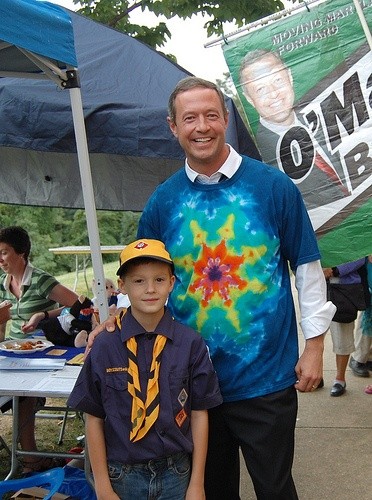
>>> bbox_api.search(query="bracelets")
[41,311,48,321]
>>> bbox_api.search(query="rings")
[312,385,317,389]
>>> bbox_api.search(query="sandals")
[20,458,66,479]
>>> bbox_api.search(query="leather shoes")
[330,382,346,396]
[349,356,369,377]
[365,361,372,371]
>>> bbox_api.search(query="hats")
[116,239,173,276]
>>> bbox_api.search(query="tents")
[0,0,263,325]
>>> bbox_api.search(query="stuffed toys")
[69,279,119,348]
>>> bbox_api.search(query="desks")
[49,245,130,292]
[0,345,97,492]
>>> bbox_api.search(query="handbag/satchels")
[326,283,371,323]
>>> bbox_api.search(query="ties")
[115,309,167,444]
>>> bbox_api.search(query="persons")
[0,226,80,474]
[314,254,372,397]
[67,239,223,500]
[83,76,337,500]
[238,49,352,211]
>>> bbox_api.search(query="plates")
[0,339,52,354]
